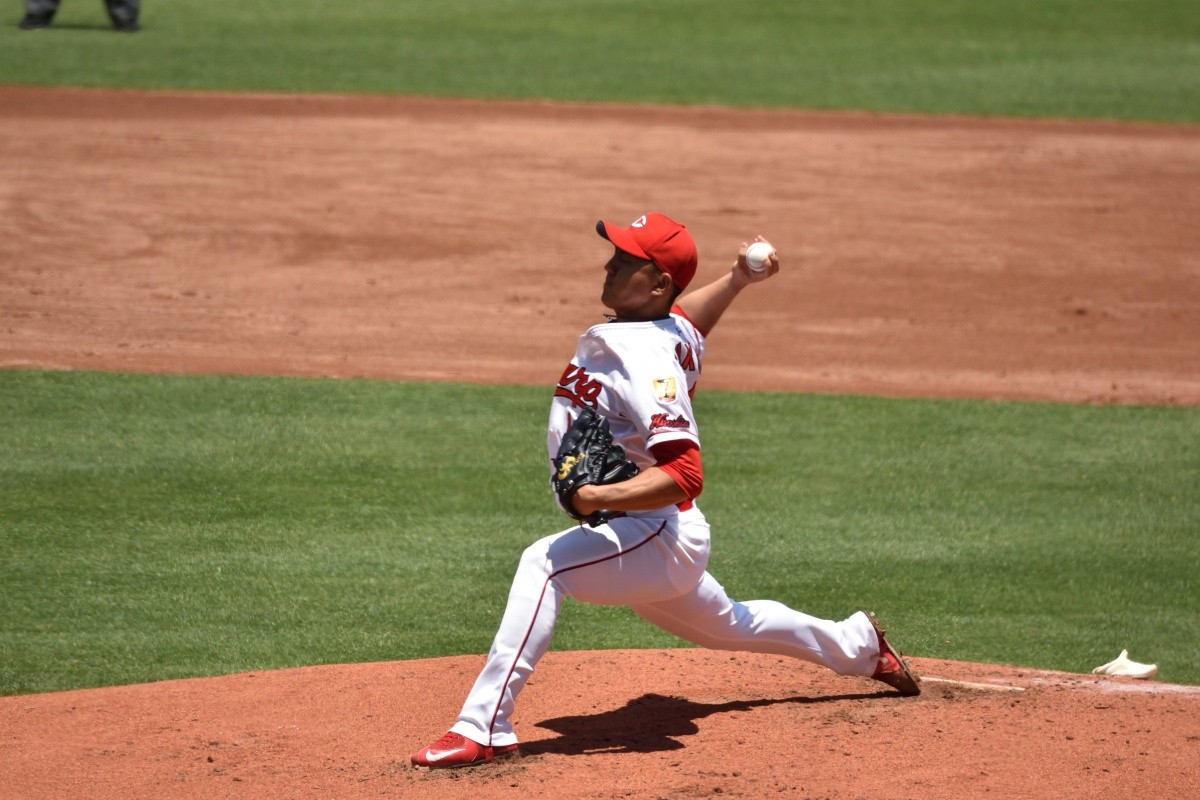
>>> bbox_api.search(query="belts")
[676,500,694,511]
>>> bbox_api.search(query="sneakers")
[410,731,520,770]
[861,610,921,696]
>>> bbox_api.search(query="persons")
[411,212,921,771]
[19,0,143,33]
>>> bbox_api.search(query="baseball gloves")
[554,407,641,526]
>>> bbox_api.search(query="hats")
[596,212,699,292]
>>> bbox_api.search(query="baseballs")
[747,242,775,272]
